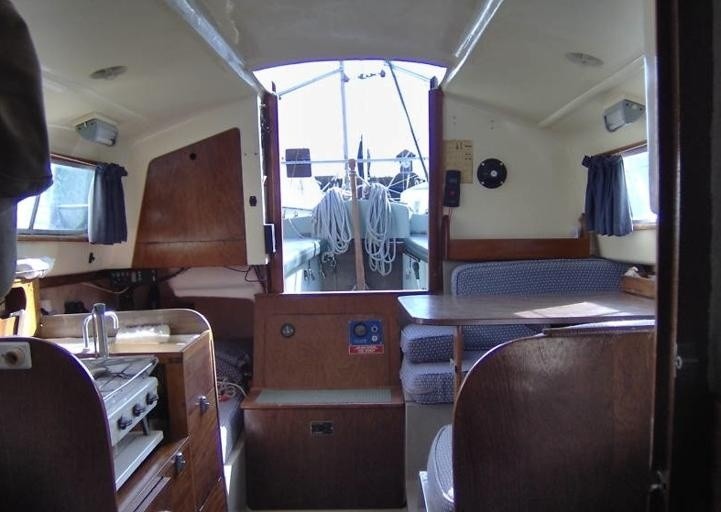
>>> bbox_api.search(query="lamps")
[76,119,117,146]
[603,99,646,132]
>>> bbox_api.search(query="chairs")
[41,307,255,511]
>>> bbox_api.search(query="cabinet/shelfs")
[0,330,227,512]
